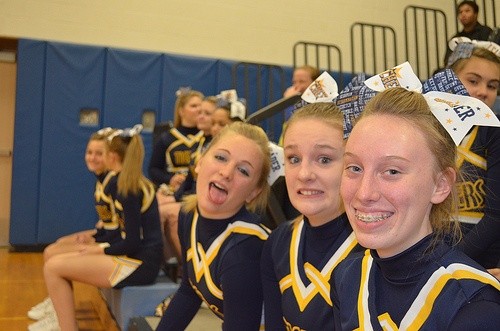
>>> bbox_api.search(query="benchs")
[98,271,183,331]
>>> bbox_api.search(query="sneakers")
[28,297,55,319]
[28,311,61,331]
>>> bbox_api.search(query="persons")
[148,89,248,282]
[260,102,363,331]
[27,127,164,331]
[283,65,321,99]
[429,37,500,282]
[154,122,274,331]
[331,86,500,331]
[432,0,493,78]
